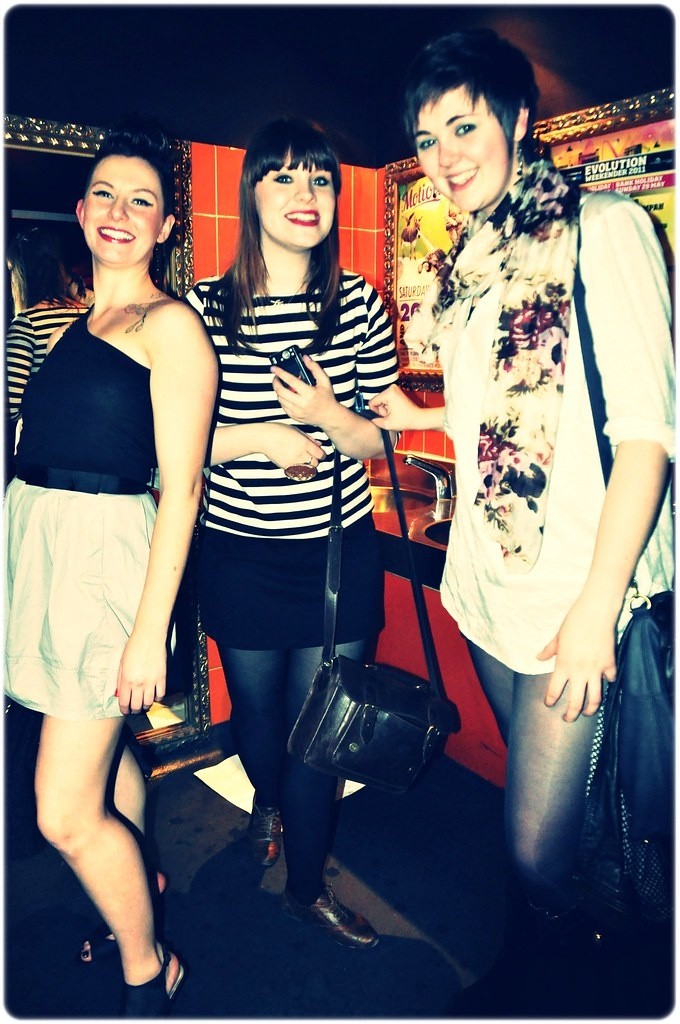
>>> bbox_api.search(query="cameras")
[269,345,316,395]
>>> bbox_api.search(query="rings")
[284,458,318,482]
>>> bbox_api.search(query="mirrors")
[5,113,224,797]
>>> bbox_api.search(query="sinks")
[423,519,453,546]
[370,486,435,513]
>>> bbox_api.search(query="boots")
[438,885,592,1017]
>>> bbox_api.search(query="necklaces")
[266,281,304,308]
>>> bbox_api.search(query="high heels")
[117,942,185,1018]
[79,873,168,963]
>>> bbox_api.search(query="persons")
[183,115,402,951]
[368,31,675,1020]
[5,140,221,1017]
[7,225,95,447]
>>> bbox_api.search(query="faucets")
[403,454,453,498]
[425,498,454,520]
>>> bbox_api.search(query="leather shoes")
[247,790,281,865]
[281,884,379,949]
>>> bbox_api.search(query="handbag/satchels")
[573,591,673,929]
[286,655,462,793]
